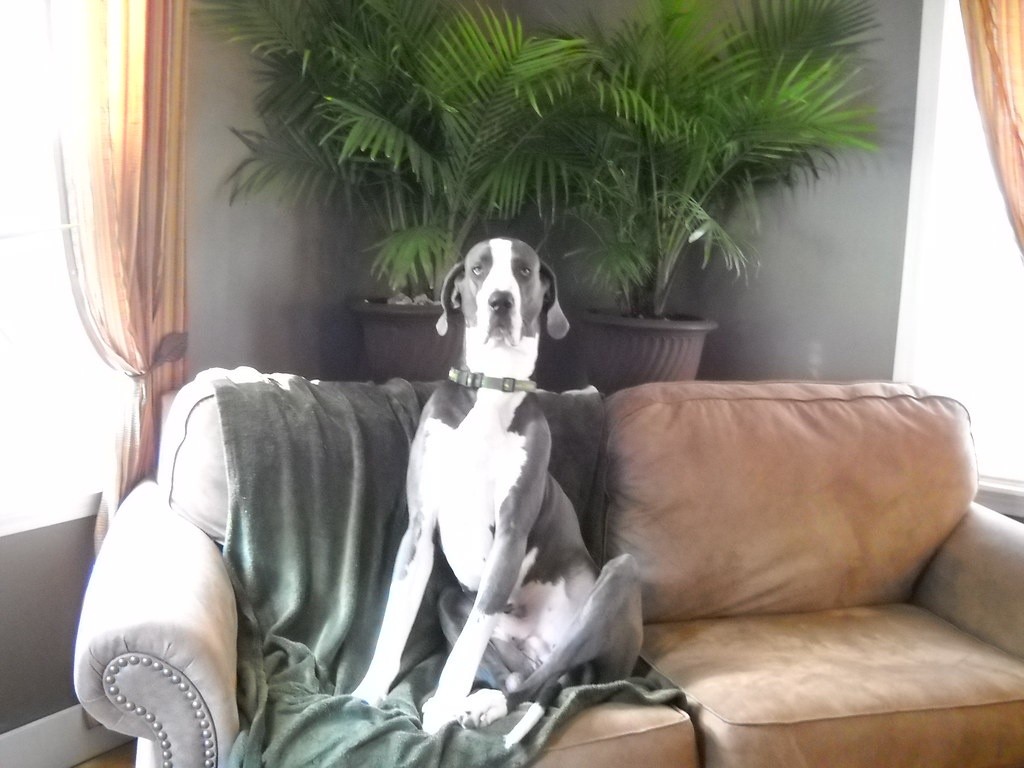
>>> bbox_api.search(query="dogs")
[349,239,645,737]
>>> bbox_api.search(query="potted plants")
[189,1,885,394]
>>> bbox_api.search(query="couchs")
[74,365,1024,768]
